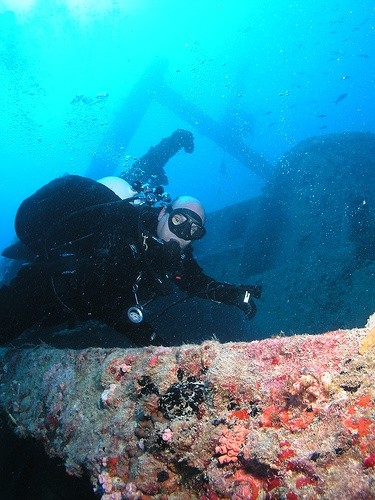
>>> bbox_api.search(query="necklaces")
[129,282,155,323]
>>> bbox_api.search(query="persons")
[0,175,261,347]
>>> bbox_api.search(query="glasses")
[167,206,206,241]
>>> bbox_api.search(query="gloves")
[234,285,263,320]
